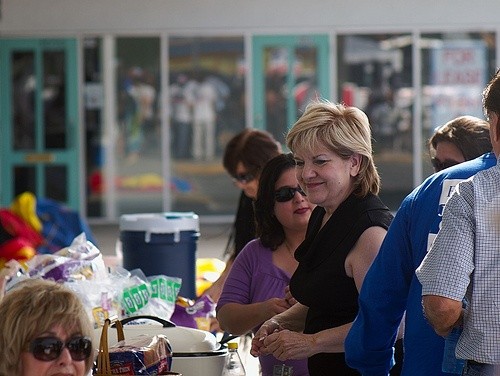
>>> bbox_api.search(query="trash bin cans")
[119,211,201,299]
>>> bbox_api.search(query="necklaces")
[284,241,294,259]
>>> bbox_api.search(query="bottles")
[222,342,246,376]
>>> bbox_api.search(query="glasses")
[233,171,257,184]
[430,156,460,173]
[21,336,92,361]
[275,183,308,202]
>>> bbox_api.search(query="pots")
[93,315,228,376]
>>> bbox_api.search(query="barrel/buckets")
[119,212,200,300]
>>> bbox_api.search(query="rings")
[257,343,263,350]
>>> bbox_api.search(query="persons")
[216,152,319,375]
[429,114,495,174]
[414,67,500,376]
[344,146,496,375]
[9,59,434,161]
[199,128,282,307]
[250,99,399,375]
[0,278,96,376]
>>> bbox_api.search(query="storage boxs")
[120,212,200,304]
